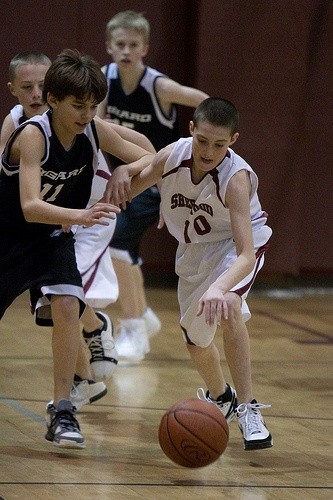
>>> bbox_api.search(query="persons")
[95,10,209,365]
[61,97,274,455]
[0,48,154,449]
[0,53,52,157]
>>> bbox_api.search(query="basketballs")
[158,398,229,469]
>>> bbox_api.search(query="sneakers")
[45,399,85,449]
[45,374,108,415]
[196,383,239,424]
[234,399,273,450]
[141,307,160,337]
[116,319,150,362]
[82,311,118,379]
